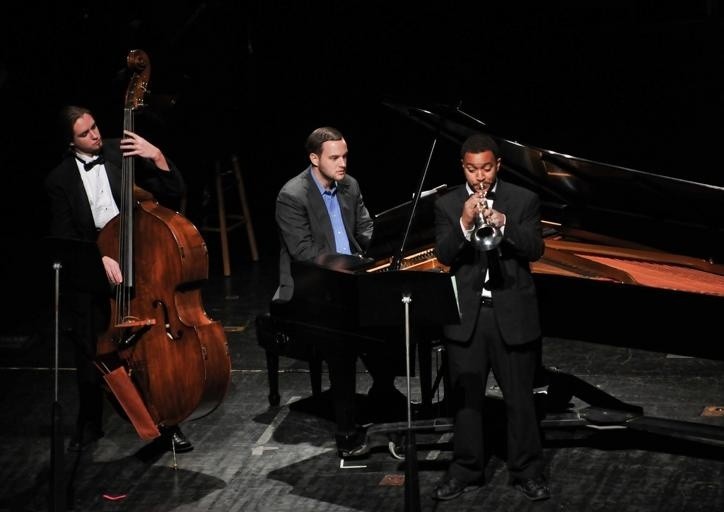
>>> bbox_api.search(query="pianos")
[314,96,723,461]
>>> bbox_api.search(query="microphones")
[412,184,448,200]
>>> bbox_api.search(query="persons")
[36,105,194,484]
[270,126,407,460]
[430,133,552,500]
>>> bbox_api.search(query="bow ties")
[84,156,105,171]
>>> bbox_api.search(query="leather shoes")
[433,475,480,500]
[514,479,549,499]
[163,425,194,452]
[369,385,407,401]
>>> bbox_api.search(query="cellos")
[91,51,231,439]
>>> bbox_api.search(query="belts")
[480,298,493,306]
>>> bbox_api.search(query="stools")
[180,154,260,276]
[255,312,406,433]
